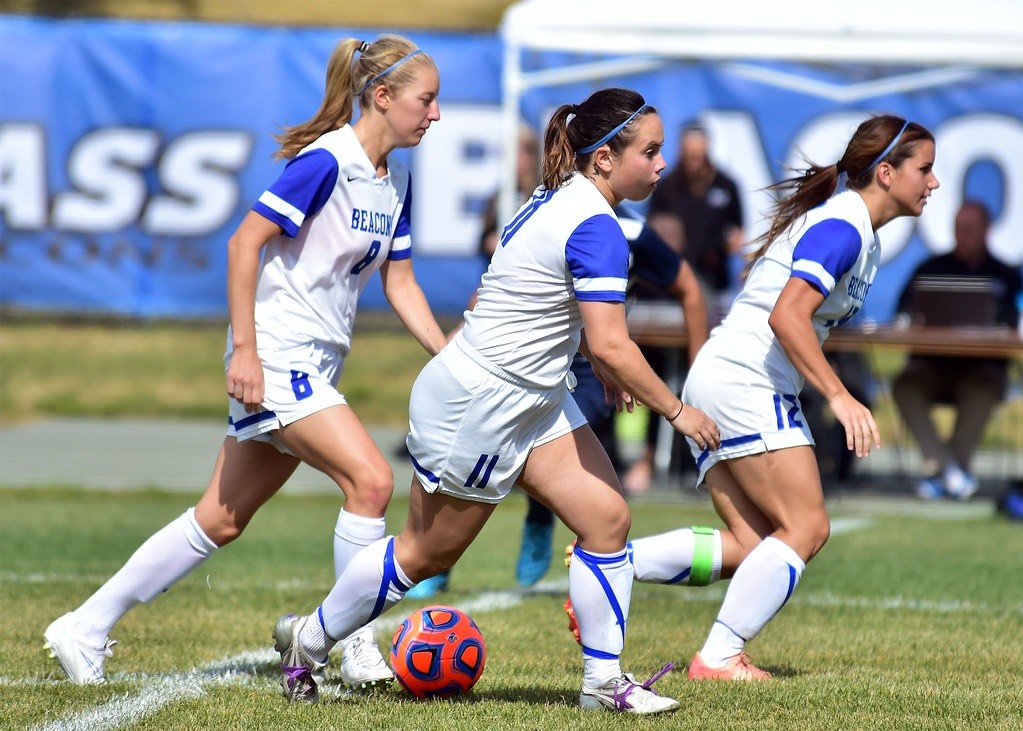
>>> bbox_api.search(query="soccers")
[390,605,486,699]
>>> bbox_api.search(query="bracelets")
[666,401,684,422]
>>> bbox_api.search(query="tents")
[499,1,1023,241]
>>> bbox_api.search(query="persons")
[476,123,540,257]
[563,114,940,681]
[44,35,447,690]
[898,203,1023,500]
[406,203,708,599]
[650,124,742,290]
[272,88,721,715]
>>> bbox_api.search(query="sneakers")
[42,611,118,687]
[578,663,680,715]
[688,651,772,681]
[564,540,581,644]
[272,614,330,706]
[341,637,394,691]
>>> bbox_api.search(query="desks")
[623,307,1023,363]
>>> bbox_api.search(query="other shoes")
[941,463,981,499]
[622,453,655,492]
[918,475,943,500]
[408,572,448,597]
[517,512,554,587]
